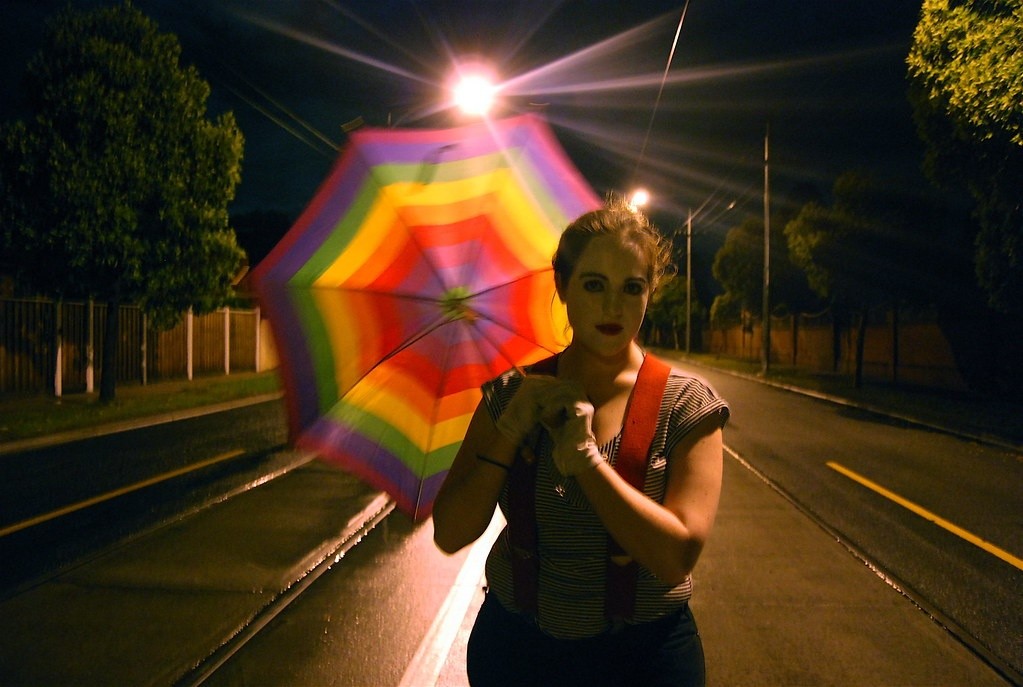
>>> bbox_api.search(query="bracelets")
[474,451,515,476]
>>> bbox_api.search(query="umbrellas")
[249,111,608,524]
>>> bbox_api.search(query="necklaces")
[550,471,574,498]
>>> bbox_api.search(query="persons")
[430,208,730,687]
